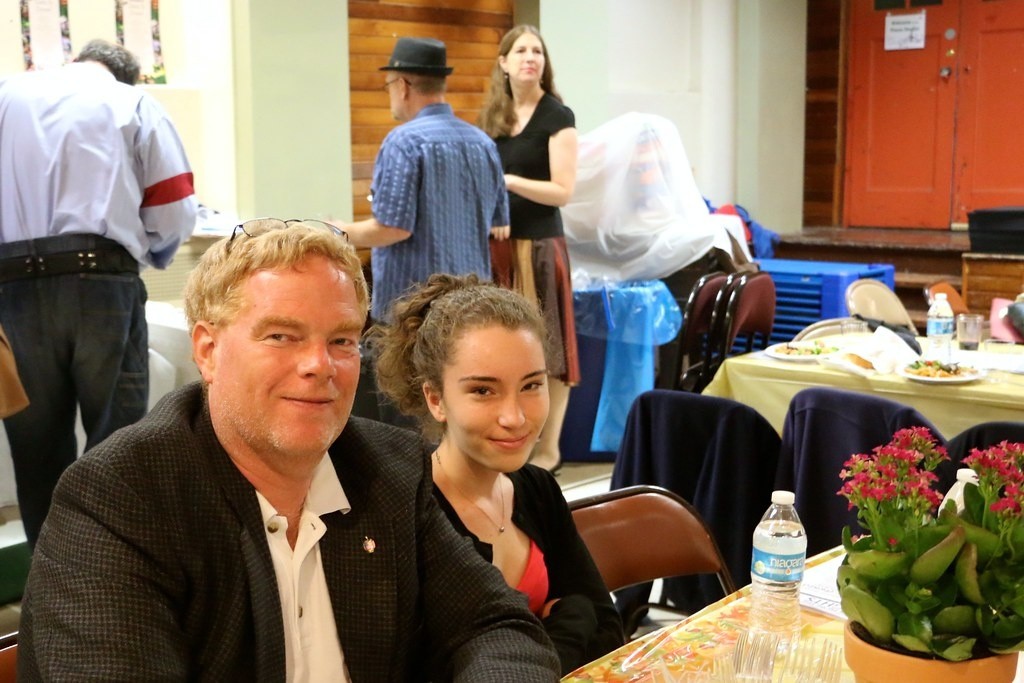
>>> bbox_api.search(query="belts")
[0,248,139,285]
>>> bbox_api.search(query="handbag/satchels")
[0,325,30,418]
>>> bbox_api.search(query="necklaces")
[435,450,505,532]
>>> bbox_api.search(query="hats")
[378,39,456,76]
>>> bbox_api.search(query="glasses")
[382,75,411,94]
[227,219,349,256]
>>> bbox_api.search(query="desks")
[559,542,1024,683]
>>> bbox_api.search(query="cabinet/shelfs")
[833,0,1024,235]
[774,225,970,334]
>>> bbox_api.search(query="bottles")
[938,469,980,519]
[927,292,954,344]
[747,491,807,652]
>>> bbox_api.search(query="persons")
[15,222,561,683]
[336,38,508,326]
[374,275,623,673]
[478,28,581,474]
[1,40,197,549]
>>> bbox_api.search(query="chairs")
[968,207,1024,254]
[610,389,782,610]
[924,279,987,330]
[661,238,776,395]
[963,251,1024,322]
[565,483,736,645]
[783,386,948,564]
[699,335,1024,442]
[929,422,1024,516]
[789,317,896,343]
[844,277,920,337]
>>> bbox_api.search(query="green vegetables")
[808,340,839,354]
[907,360,943,369]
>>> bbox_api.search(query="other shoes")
[550,456,561,476]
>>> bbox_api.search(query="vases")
[842,618,1019,683]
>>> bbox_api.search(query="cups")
[840,319,868,337]
[985,339,1015,383]
[956,313,984,350]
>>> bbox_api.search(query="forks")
[713,632,843,683]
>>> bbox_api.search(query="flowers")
[833,425,1024,683]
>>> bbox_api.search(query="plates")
[766,342,842,361]
[894,360,980,384]
